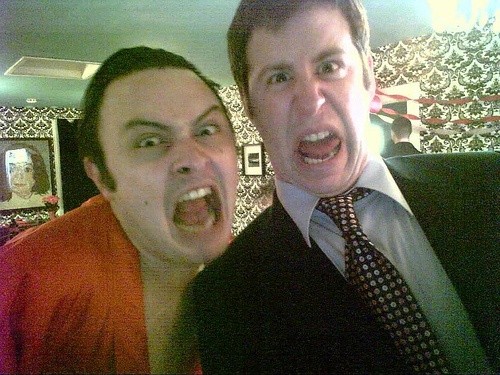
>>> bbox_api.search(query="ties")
[315,188,451,374]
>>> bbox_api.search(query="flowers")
[42,196,60,212]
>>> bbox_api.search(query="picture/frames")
[0,137,57,216]
[242,143,265,176]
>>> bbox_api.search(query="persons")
[380,117,422,159]
[191,0,500,375]
[0,47,239,375]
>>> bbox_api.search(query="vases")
[49,211,56,221]
